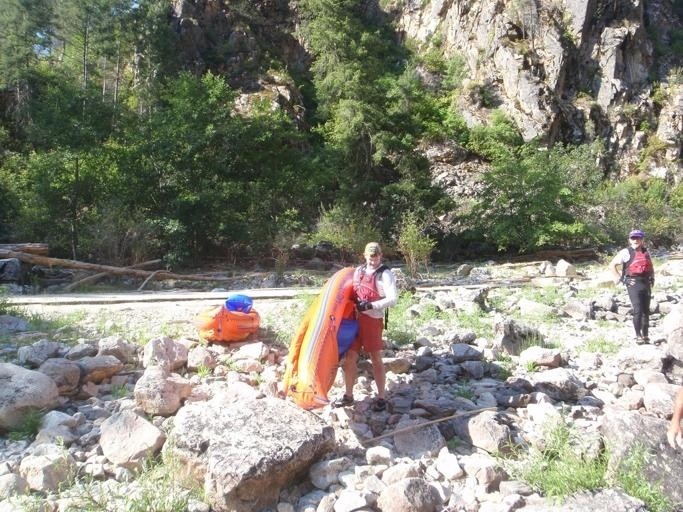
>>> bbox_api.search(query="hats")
[365,242,381,255]
[629,230,644,238]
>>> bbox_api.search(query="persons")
[607,229,656,345]
[332,241,399,411]
[665,385,683,451]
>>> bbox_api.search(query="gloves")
[356,300,373,311]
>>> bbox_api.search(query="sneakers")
[335,394,355,408]
[636,336,651,345]
[375,398,386,411]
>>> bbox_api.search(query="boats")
[284,267,360,410]
[198,302,261,345]
